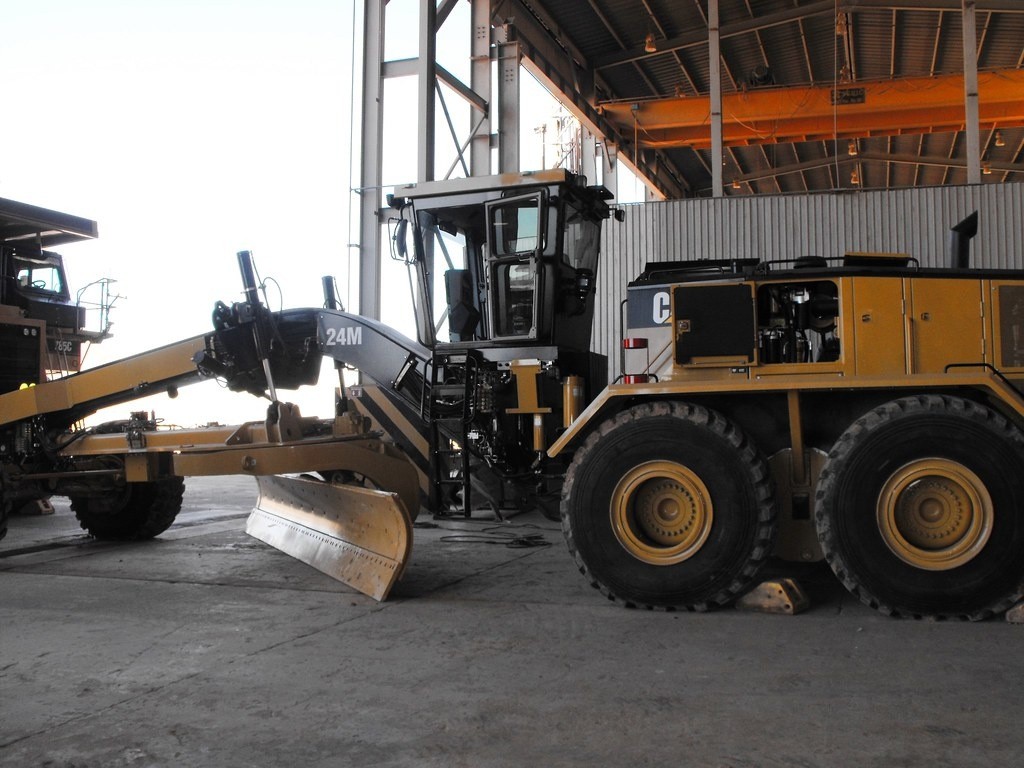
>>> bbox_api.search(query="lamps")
[750,65,772,85]
[982,163,991,174]
[848,140,857,155]
[995,130,1005,146]
[850,169,859,183]
[733,177,741,188]
[674,84,685,98]
[836,9,847,35]
[840,64,850,84]
[644,15,656,51]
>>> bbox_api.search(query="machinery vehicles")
[0,168,1023,625]
[0,198,425,603]
